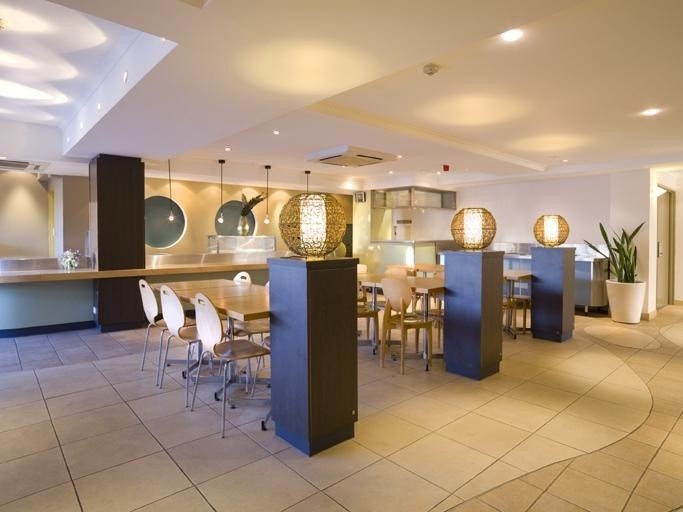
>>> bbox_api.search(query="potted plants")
[583,221,650,325]
[236,192,270,236]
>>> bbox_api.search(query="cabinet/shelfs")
[532,247,577,344]
[264,258,360,458]
[443,251,505,379]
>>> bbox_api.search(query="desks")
[149,278,270,378]
[496,252,609,316]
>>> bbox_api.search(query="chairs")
[232,281,270,347]
[356,264,530,375]
[159,283,228,408]
[233,270,251,283]
[139,278,201,385]
[190,293,270,438]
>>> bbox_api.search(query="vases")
[65,261,73,275]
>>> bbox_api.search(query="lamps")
[532,214,570,246]
[278,193,347,255]
[263,166,271,225]
[217,157,225,224]
[168,159,175,222]
[449,208,498,249]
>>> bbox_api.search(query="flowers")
[58,249,82,274]
